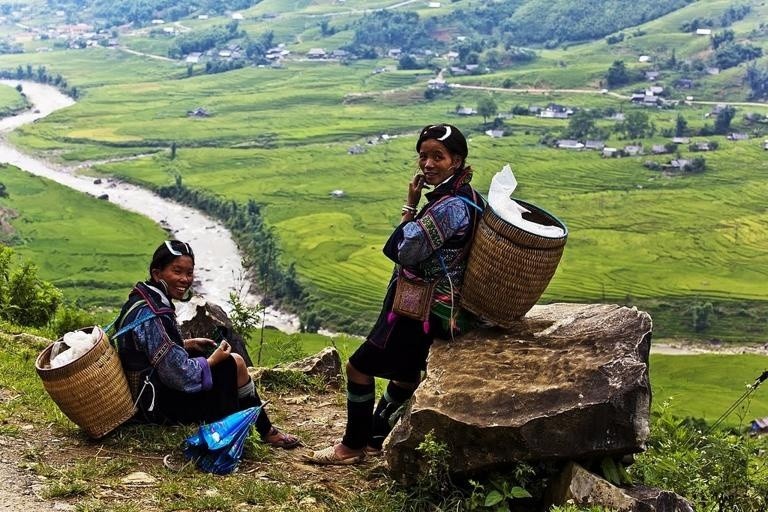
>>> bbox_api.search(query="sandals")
[256,427,302,447]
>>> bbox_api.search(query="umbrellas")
[163,398,270,475]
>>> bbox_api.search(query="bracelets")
[400,204,417,216]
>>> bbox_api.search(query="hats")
[416,122,468,156]
[149,238,195,277]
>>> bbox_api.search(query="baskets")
[33,323,140,438]
[461,196,569,331]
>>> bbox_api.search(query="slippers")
[304,442,361,464]
[365,447,383,456]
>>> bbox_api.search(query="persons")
[299,122,487,466]
[113,238,300,449]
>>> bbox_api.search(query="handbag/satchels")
[393,276,435,321]
